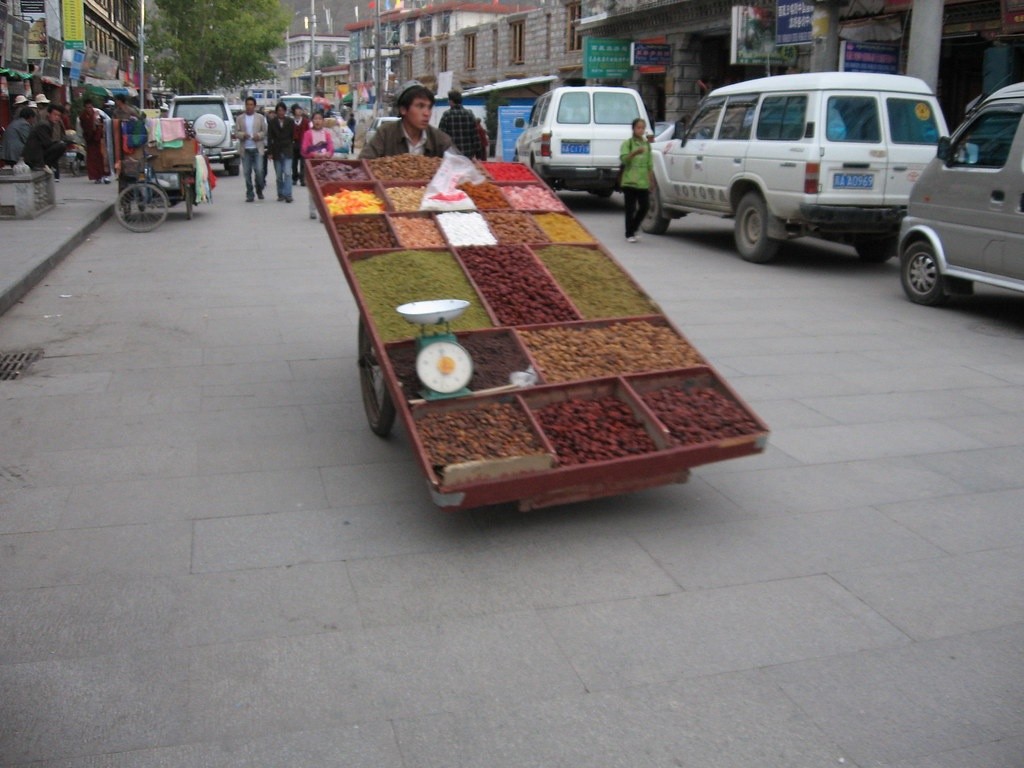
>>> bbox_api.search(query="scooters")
[66,129,87,177]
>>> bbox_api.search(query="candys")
[322,187,388,216]
[500,181,562,209]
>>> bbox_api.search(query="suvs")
[166,94,241,177]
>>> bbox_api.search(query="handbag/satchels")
[421,146,484,211]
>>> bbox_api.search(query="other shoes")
[43,164,56,175]
[95,179,101,184]
[627,235,640,242]
[301,180,305,186]
[246,198,254,202]
[285,194,293,203]
[256,190,264,199]
[277,195,285,201]
[310,213,316,218]
[54,178,59,182]
[104,179,111,184]
[292,180,297,185]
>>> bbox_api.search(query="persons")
[235,96,335,223]
[346,113,356,153]
[476,117,488,162]
[438,90,482,162]
[0,94,69,183]
[357,78,453,161]
[79,99,112,185]
[619,118,655,243]
[113,92,138,119]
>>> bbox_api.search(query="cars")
[365,117,403,147]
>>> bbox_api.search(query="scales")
[392,298,473,400]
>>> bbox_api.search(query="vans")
[278,92,315,125]
[639,72,950,266]
[512,84,653,200]
[896,81,1024,308]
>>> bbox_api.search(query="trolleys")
[357,306,397,438]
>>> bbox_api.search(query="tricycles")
[114,117,199,232]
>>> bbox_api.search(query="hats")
[35,94,50,103]
[394,79,425,103]
[14,96,28,104]
[27,100,37,107]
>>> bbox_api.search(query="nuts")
[519,321,710,384]
